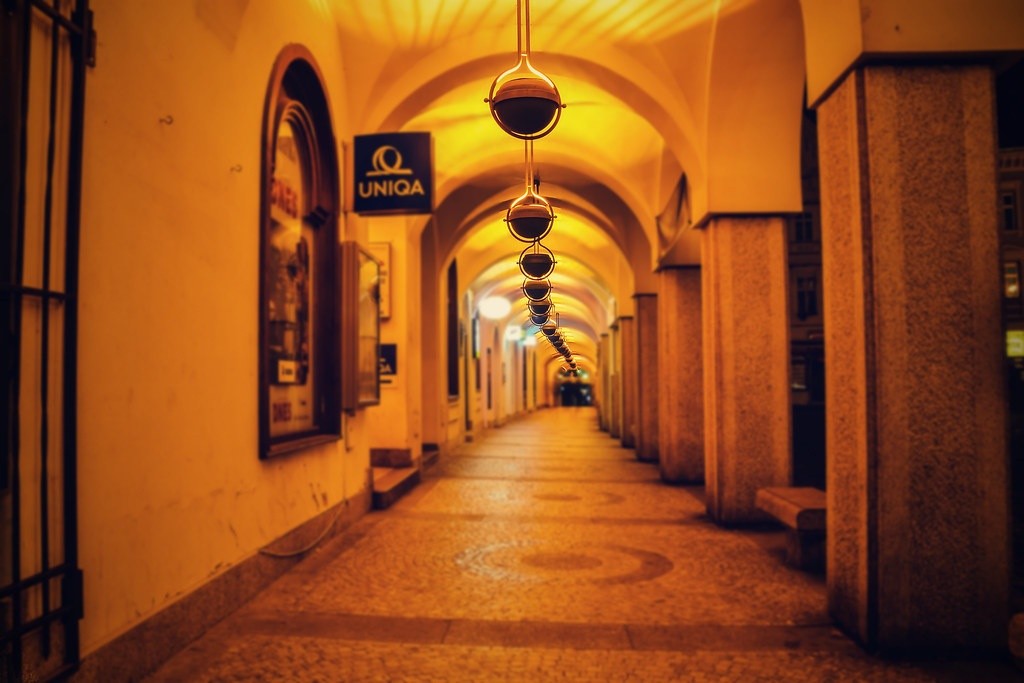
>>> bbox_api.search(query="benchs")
[754,487,826,574]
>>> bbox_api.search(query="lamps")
[484,0,584,380]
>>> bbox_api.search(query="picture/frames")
[357,241,393,322]
